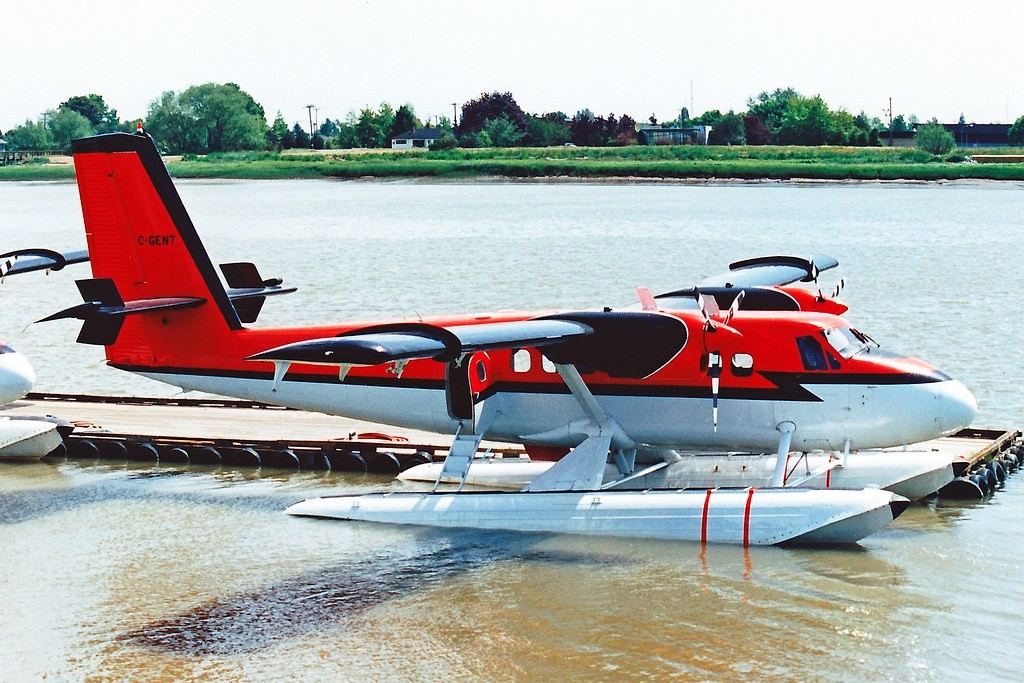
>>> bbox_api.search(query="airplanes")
[1,249,90,462]
[31,122,979,546]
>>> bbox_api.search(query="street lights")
[305,104,315,149]
[314,108,319,132]
[450,102,456,126]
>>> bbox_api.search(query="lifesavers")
[68,439,434,475]
[939,443,1023,500]
